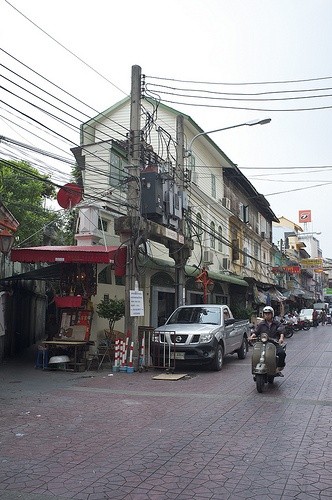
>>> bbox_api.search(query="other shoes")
[277,372,284,377]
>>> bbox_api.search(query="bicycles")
[249,315,312,346]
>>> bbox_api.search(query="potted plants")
[96,295,126,364]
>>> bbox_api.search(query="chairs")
[84,341,112,372]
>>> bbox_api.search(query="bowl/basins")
[48,355,71,364]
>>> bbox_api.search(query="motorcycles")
[246,333,287,392]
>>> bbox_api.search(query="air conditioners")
[203,251,215,265]
[222,197,231,211]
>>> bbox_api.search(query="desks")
[41,339,88,372]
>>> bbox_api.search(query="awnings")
[11,243,126,276]
[248,277,318,303]
[3,262,95,284]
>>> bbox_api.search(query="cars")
[300,308,316,326]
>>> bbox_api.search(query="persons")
[280,305,328,330]
[246,306,286,377]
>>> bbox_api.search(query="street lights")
[195,267,215,304]
[176,117,271,305]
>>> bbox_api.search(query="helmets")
[261,306,274,317]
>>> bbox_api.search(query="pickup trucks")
[150,304,252,372]
